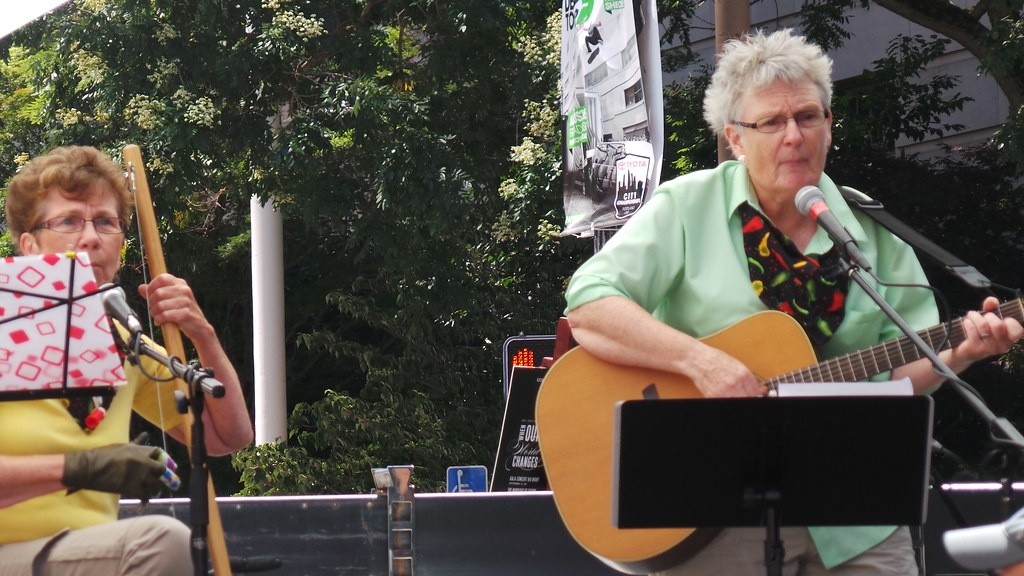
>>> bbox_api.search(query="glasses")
[29,215,127,234]
[731,109,830,134]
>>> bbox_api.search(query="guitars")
[532,296,1024,576]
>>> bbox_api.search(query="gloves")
[62,432,182,506]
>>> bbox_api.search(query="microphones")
[98,283,143,338]
[795,186,872,273]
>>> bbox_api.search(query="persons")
[0,144,253,576]
[564,27,1024,576]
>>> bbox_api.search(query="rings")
[980,333,992,339]
